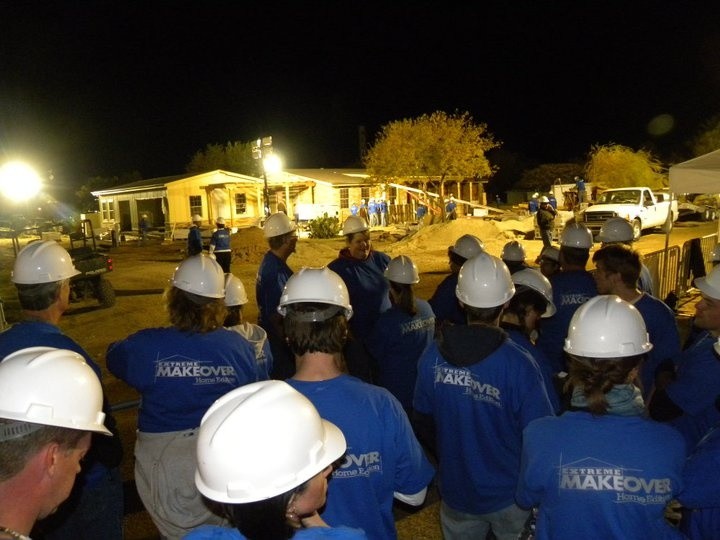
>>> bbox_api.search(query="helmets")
[264,213,297,237]
[0,346,113,436]
[511,268,556,318]
[195,380,347,504]
[278,267,352,321]
[707,243,720,262]
[452,235,484,259]
[192,215,202,222]
[11,241,81,284]
[575,177,579,181]
[694,264,720,300]
[532,191,553,202]
[596,219,634,243]
[216,217,226,224]
[501,241,526,262]
[559,223,594,248]
[456,253,516,308]
[564,295,653,358]
[343,215,370,237]
[385,255,419,284]
[173,254,225,299]
[223,273,249,307]
[542,247,560,263]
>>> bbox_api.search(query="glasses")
[541,259,545,264]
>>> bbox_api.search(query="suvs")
[12,219,116,308]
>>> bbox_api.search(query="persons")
[501,268,560,412]
[0,177,720,540]
[257,213,296,380]
[187,214,203,256]
[365,255,435,413]
[209,217,231,273]
[546,219,597,347]
[327,215,393,369]
[426,234,484,328]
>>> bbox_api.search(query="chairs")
[69,233,90,253]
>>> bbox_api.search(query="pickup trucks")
[585,187,680,240]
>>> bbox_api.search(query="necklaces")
[0,527,32,540]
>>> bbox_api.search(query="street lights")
[250,136,285,218]
